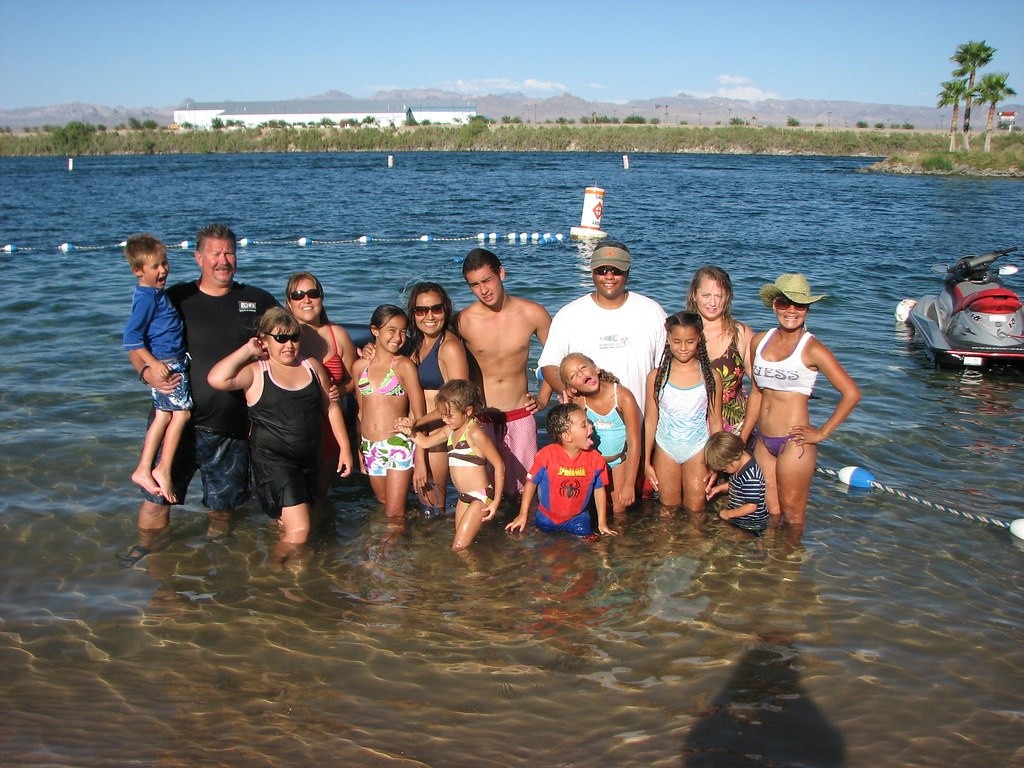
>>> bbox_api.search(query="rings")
[802,436,804,439]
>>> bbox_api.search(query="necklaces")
[596,289,626,309]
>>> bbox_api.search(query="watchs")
[139,364,149,385]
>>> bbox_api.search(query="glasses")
[290,289,321,300]
[593,266,625,276]
[773,298,810,311]
[264,332,300,343]
[414,304,445,316]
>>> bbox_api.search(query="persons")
[352,248,553,549]
[286,272,357,474]
[505,403,619,538]
[559,353,640,513]
[538,240,668,494]
[123,224,285,530]
[644,312,723,512]
[704,431,770,537]
[686,265,759,455]
[207,307,353,544]
[740,275,861,526]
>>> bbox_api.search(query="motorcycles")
[908,245,1024,370]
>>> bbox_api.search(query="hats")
[589,246,632,273]
[759,273,826,308]
